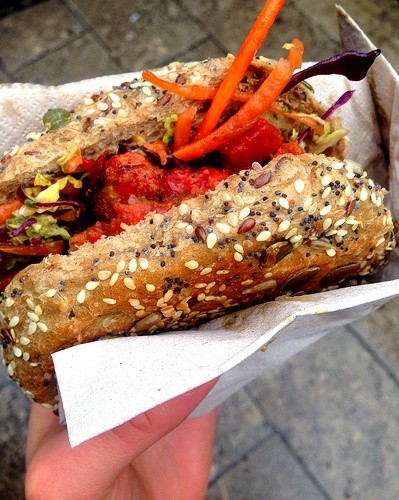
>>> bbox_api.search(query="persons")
[0,358,219,500]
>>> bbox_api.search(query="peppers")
[190,0,287,143]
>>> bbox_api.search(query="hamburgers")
[0,50,396,407]
